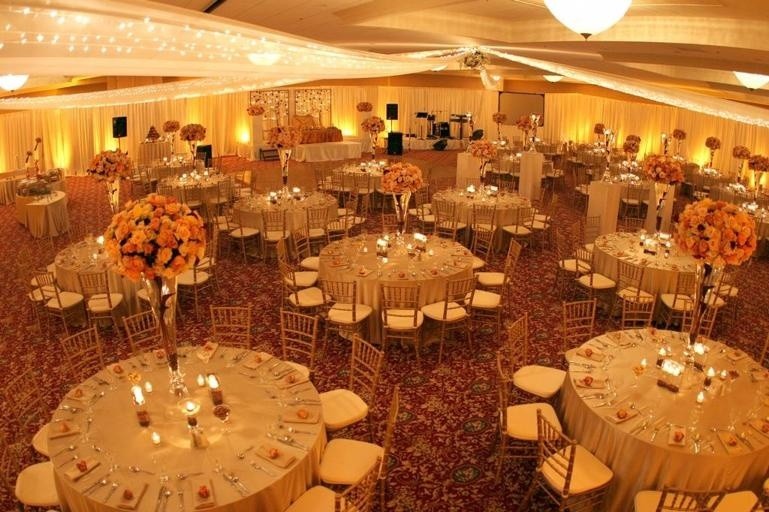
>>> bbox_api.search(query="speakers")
[197,145,212,167]
[388,133,402,155]
[112,117,127,138]
[386,104,398,120]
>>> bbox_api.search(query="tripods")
[416,119,425,141]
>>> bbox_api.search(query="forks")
[650,425,662,442]
[176,486,185,512]
[103,481,121,502]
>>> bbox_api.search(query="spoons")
[82,478,109,498]
[162,488,172,512]
[229,472,249,494]
[50,445,78,459]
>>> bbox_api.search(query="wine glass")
[606,226,675,266]
[599,322,769,433]
[336,228,450,278]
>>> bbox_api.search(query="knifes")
[221,474,246,498]
[80,473,110,494]
[153,487,164,512]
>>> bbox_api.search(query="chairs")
[274,198,522,365]
[91,146,766,267]
[3,306,401,510]
[27,226,216,338]
[492,296,769,510]
[3,154,68,237]
[557,214,741,338]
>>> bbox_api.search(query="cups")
[77,346,289,480]
[66,231,105,263]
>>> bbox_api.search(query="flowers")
[491,112,725,147]
[457,47,493,73]
[162,98,387,146]
